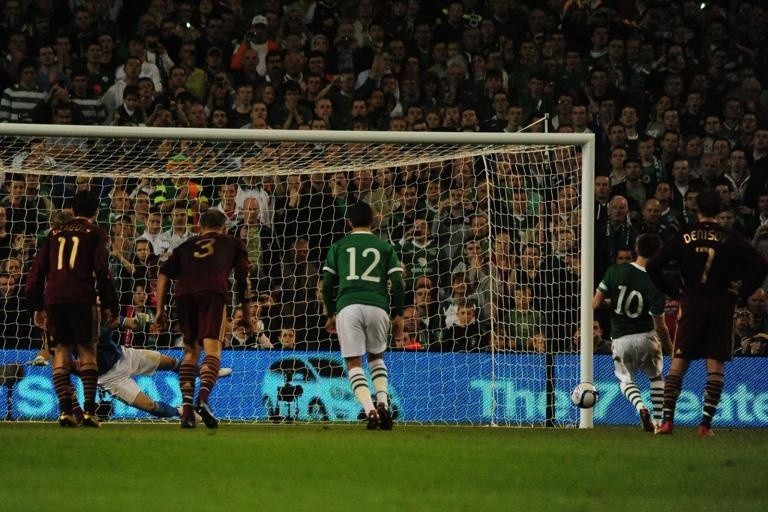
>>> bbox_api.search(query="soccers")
[0,364,24,386]
[572,382,598,408]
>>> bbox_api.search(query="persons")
[33,301,232,422]
[156,211,255,428]
[1,0,767,355]
[322,202,405,429]
[660,189,767,437]
[26,190,121,426]
[593,234,673,432]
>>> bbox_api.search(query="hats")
[207,46,223,56]
[751,329,768,344]
[115,214,132,223]
[311,34,330,51]
[252,15,268,26]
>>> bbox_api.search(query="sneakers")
[698,422,715,438]
[217,367,232,378]
[179,405,219,429]
[367,402,392,430]
[59,406,100,428]
[638,405,674,434]
